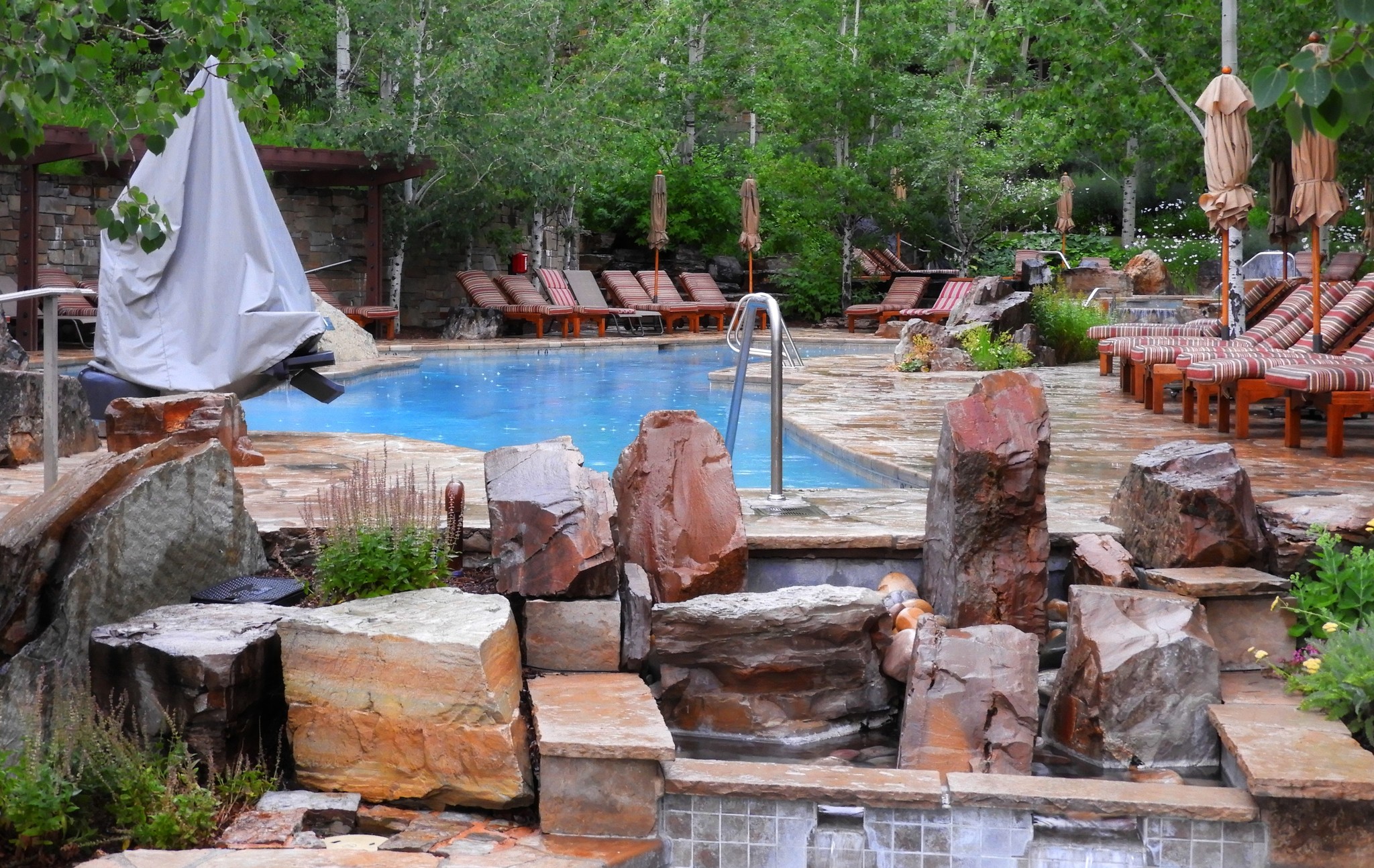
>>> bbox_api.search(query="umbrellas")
[647,169,668,328]
[1054,172,1076,269]
[1266,156,1306,279]
[739,176,763,294]
[1194,65,1256,328]
[889,166,907,260]
[1358,144,1374,251]
[1289,30,1349,336]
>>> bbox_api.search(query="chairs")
[306,274,399,340]
[1085,250,1374,458]
[844,250,1043,334]
[456,270,767,339]
[0,268,98,349]
